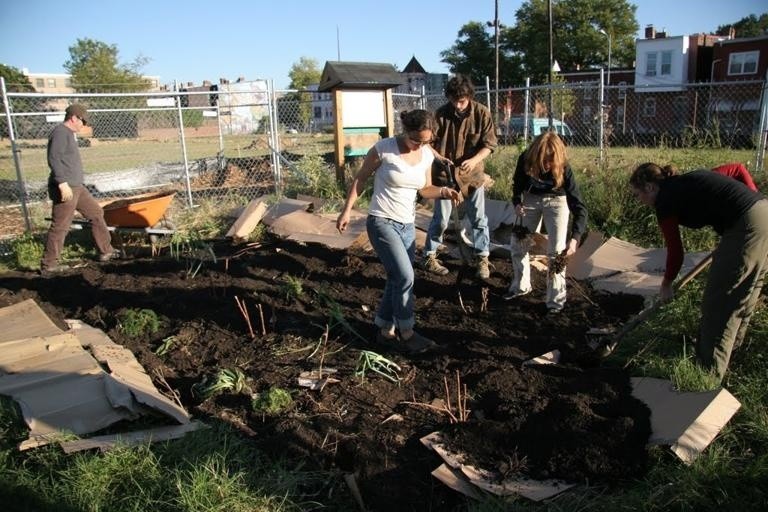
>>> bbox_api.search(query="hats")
[65,104,89,122]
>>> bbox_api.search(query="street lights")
[486,0,507,134]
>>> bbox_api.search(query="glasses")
[77,116,88,127]
[407,132,436,145]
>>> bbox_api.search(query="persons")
[42,104,124,272]
[629,160,768,388]
[422,79,498,279]
[338,108,461,352]
[504,133,588,315]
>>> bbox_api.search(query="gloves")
[58,181,74,202]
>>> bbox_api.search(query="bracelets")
[438,186,446,200]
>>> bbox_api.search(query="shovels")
[592,254,712,359]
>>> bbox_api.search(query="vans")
[510,118,572,136]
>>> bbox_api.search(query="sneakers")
[547,307,561,319]
[501,288,533,300]
[418,252,450,277]
[474,256,491,280]
[42,264,70,275]
[98,248,122,261]
[400,330,437,354]
[375,332,400,351]
